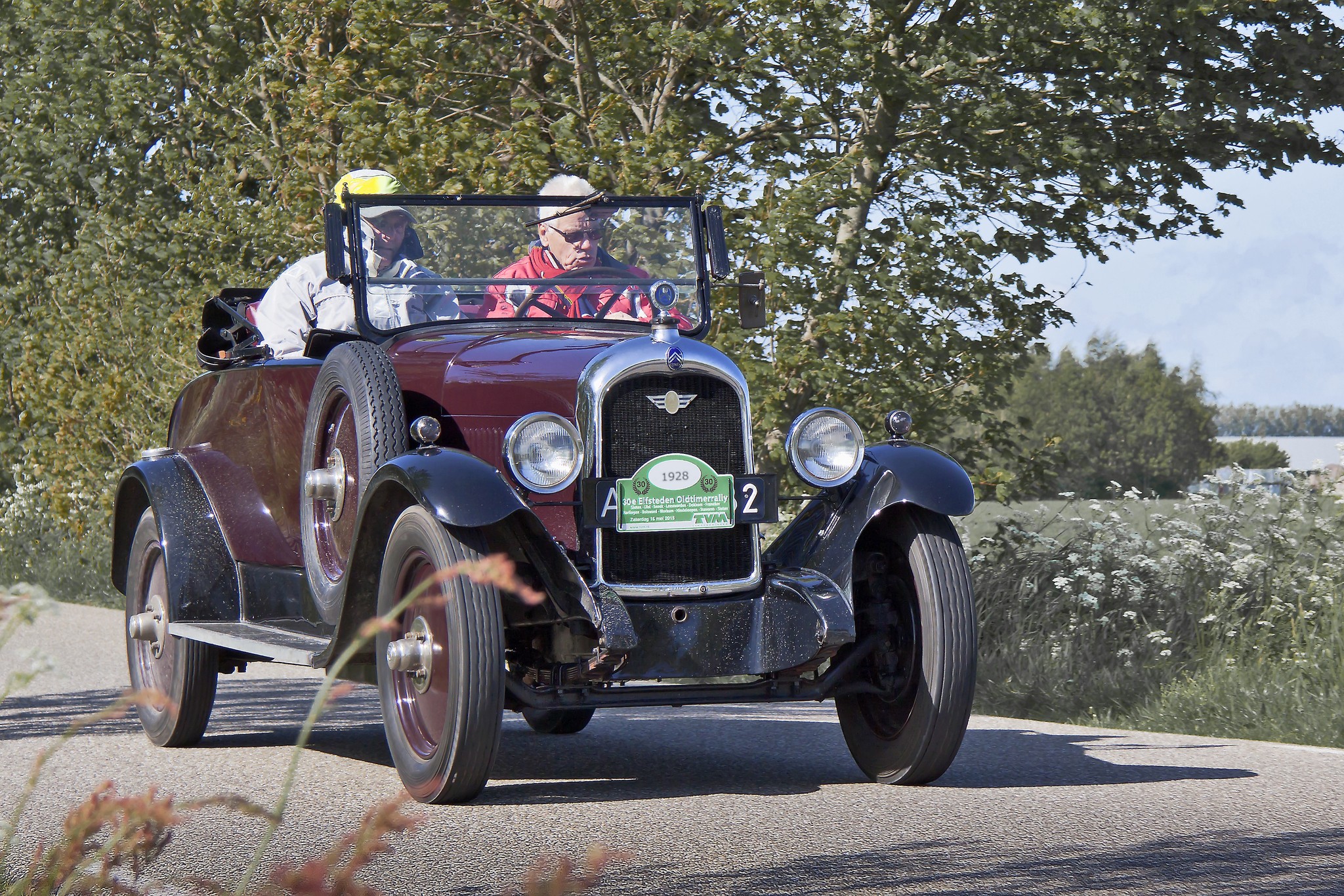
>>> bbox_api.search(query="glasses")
[545,223,606,243]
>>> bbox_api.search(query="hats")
[333,169,417,224]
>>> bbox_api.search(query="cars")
[110,177,981,808]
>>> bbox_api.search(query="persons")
[474,175,669,322]
[256,169,461,360]
